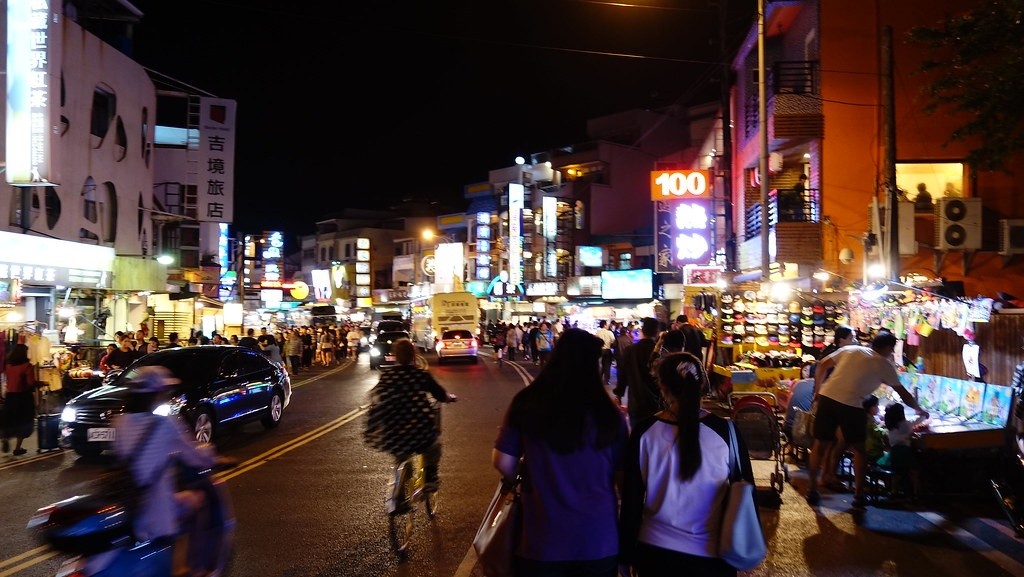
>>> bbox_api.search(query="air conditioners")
[934,198,982,250]
[997,219,1024,255]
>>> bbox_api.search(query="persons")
[258,322,364,376]
[0,344,45,455]
[108,365,215,577]
[99,330,160,375]
[479,315,758,577]
[187,327,258,346]
[164,332,182,349]
[783,327,928,510]
[363,338,451,492]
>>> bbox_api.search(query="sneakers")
[805,490,821,505]
[851,496,869,512]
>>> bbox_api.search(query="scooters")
[24,442,238,577]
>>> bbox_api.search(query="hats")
[126,367,181,392]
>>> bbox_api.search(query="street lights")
[421,228,456,292]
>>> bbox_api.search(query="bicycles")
[384,394,461,554]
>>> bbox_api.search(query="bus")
[408,291,479,353]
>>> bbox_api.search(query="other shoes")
[426,476,443,488]
[13,448,27,456]
[3,440,9,453]
[524,355,541,366]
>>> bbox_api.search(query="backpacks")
[522,328,530,345]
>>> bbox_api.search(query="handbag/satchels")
[473,488,518,577]
[721,420,765,569]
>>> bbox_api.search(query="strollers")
[727,391,791,493]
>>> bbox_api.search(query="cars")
[58,343,292,459]
[434,329,480,365]
[357,311,417,370]
